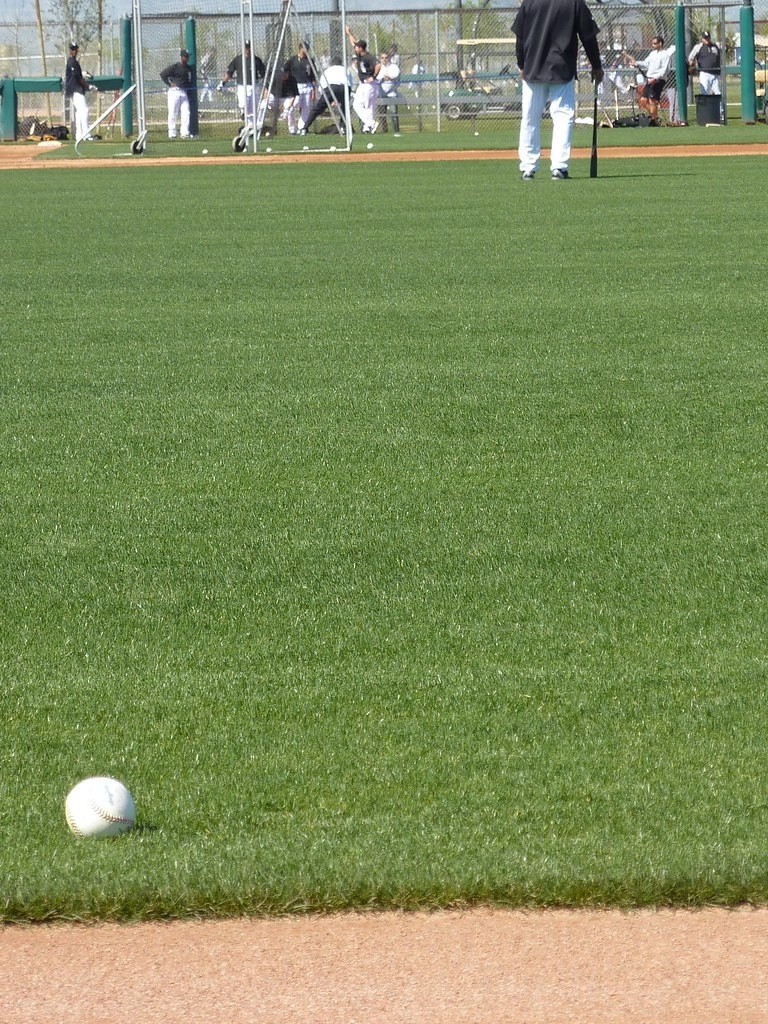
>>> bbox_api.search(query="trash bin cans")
[696,92,723,127]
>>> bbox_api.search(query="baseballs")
[474,132,479,136]
[202,149,208,154]
[266,148,272,152]
[303,146,309,150]
[367,143,373,150]
[330,146,336,152]
[62,776,136,841]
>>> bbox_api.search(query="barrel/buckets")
[694,94,721,126]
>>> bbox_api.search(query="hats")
[178,49,192,56]
[68,42,78,50]
[330,57,343,66]
[299,41,310,48]
[244,40,251,48]
[353,40,367,50]
[702,31,710,38]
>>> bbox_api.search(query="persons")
[631,32,730,126]
[199,47,217,103]
[599,36,636,103]
[217,40,266,136]
[159,49,194,139]
[65,44,99,142]
[512,0,604,181]
[267,26,401,146]
[412,58,425,90]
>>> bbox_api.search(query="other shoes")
[290,128,308,135]
[83,136,94,140]
[648,120,659,127]
[341,128,346,135]
[180,134,194,139]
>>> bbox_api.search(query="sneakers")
[522,171,536,181]
[361,122,380,135]
[551,168,573,180]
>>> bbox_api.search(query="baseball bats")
[589,80,599,178]
[40,114,55,125]
[599,102,614,129]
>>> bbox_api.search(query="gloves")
[89,84,98,92]
[216,81,226,91]
